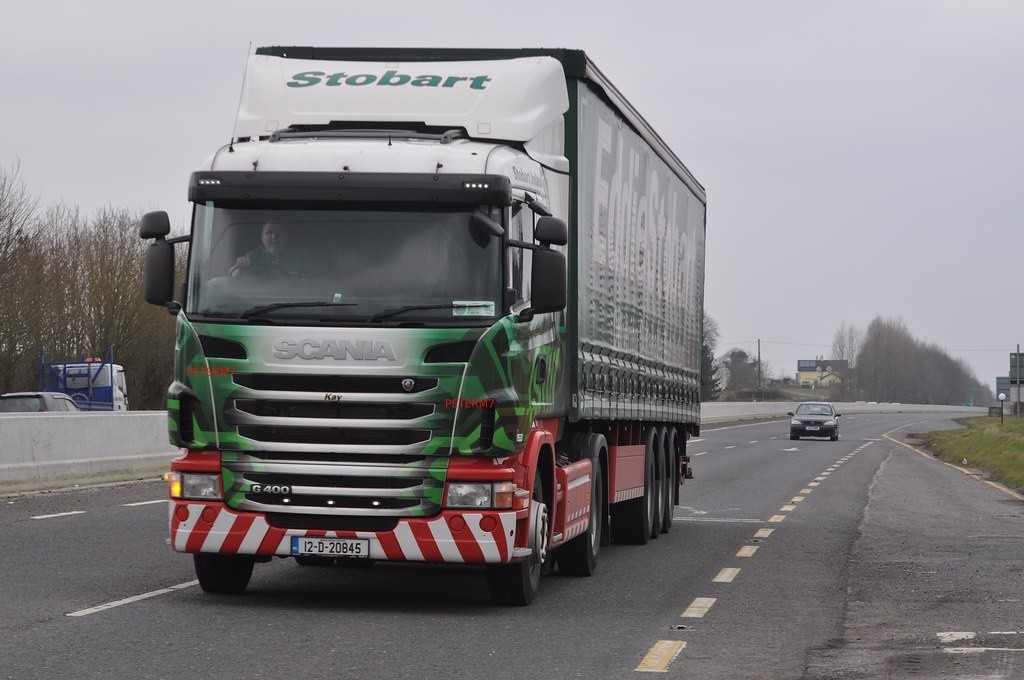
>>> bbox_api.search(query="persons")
[236,219,319,283]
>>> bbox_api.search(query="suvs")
[0,391,81,413]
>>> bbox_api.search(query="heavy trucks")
[137,41,712,609]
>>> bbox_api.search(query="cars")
[787,402,842,442]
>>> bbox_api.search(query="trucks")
[41,344,131,411]
